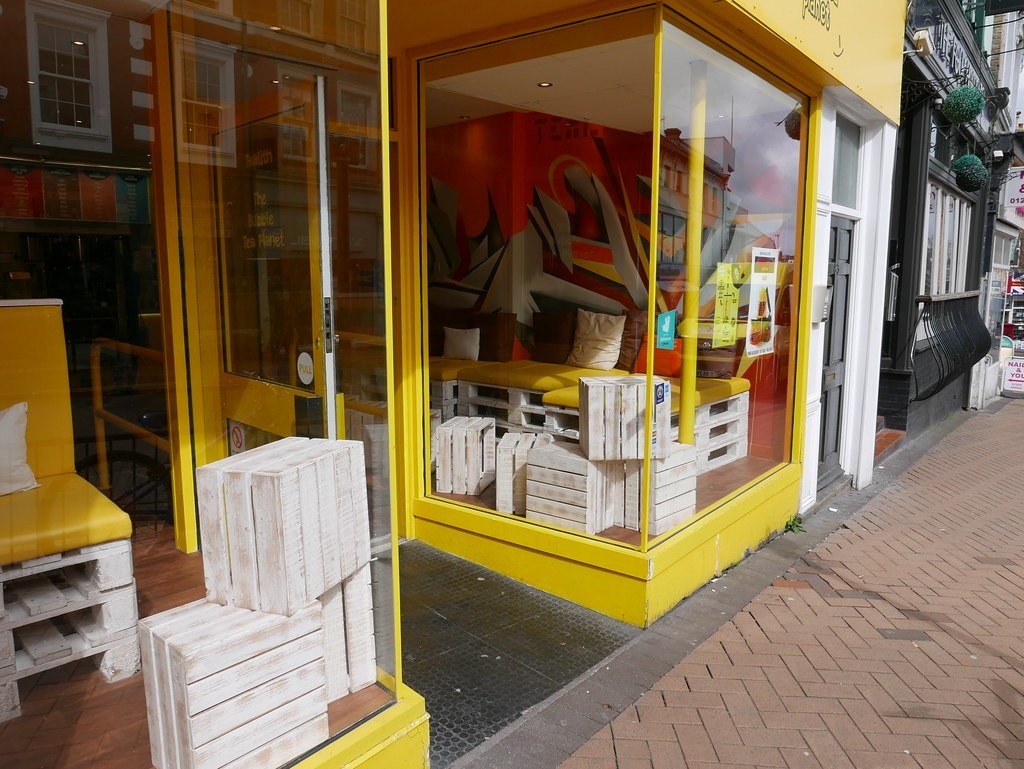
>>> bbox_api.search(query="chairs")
[1,300,140,723]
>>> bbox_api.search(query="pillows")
[0,402,41,497]
[631,332,683,378]
[697,325,736,380]
[443,327,480,360]
[564,308,626,371]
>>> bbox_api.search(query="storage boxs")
[436,376,696,537]
[138,435,375,769]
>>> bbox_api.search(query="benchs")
[327,299,751,477]
